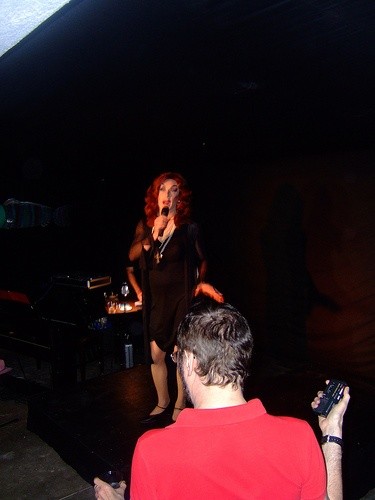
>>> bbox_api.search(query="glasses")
[171,351,178,363]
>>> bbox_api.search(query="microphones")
[159,208,168,236]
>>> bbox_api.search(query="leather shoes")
[171,399,183,423]
[144,398,172,422]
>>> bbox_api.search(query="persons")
[112,231,144,302]
[125,168,224,427]
[90,290,354,500]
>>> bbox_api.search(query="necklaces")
[149,213,177,260]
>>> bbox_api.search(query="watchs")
[318,434,347,450]
